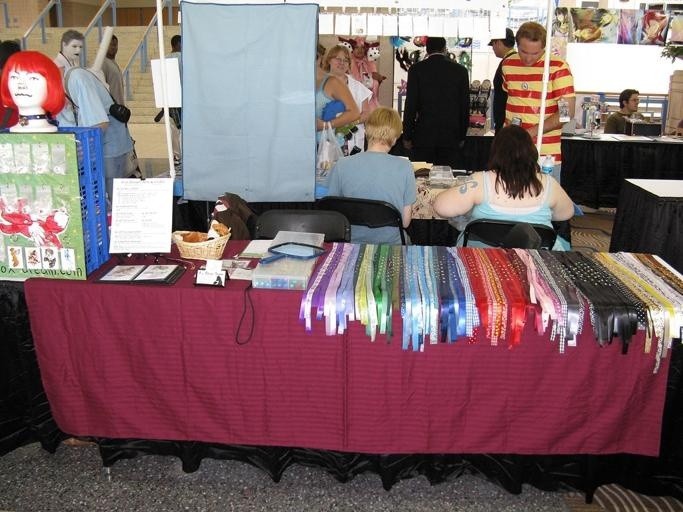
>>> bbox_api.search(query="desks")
[608,179,683,275]
[409,169,472,219]
[560,131,683,209]
[466,127,495,136]
[25,239,682,503]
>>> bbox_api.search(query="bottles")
[541,154,553,175]
[579,96,606,138]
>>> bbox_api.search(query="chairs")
[464,217,557,252]
[256,210,350,240]
[318,196,405,245]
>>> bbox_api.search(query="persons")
[164,35,181,165]
[432,124,584,251]
[501,21,575,185]
[0,28,142,204]
[315,35,417,244]
[487,28,517,137]
[604,89,645,133]
[403,36,470,168]
[676,120,683,135]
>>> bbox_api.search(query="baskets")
[172,229,232,262]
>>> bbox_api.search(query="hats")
[487,26,516,47]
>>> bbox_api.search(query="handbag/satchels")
[109,102,132,124]
